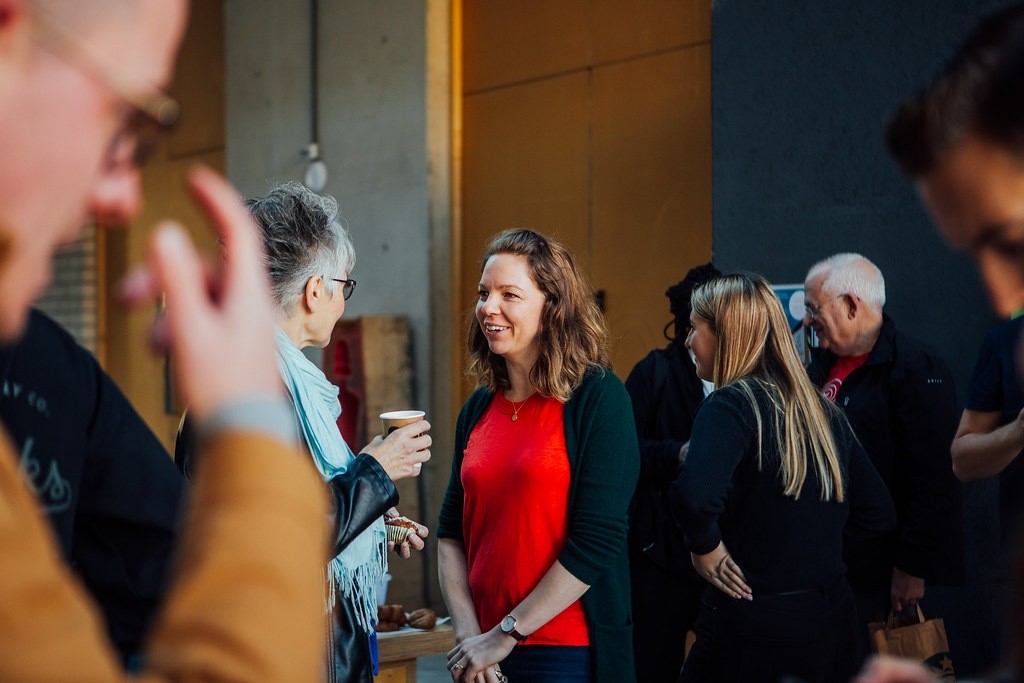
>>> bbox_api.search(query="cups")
[380,410,425,468]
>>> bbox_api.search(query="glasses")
[10,0,182,172]
[805,293,860,320]
[321,277,358,300]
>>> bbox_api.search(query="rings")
[453,662,464,670]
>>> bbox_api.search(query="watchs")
[499,614,528,642]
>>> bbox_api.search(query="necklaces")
[507,388,534,420]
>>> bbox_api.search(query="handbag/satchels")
[870,600,956,683]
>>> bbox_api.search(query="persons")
[434,229,642,683]
[0,0,330,683]
[626,0,1024,683]
[174,185,432,683]
